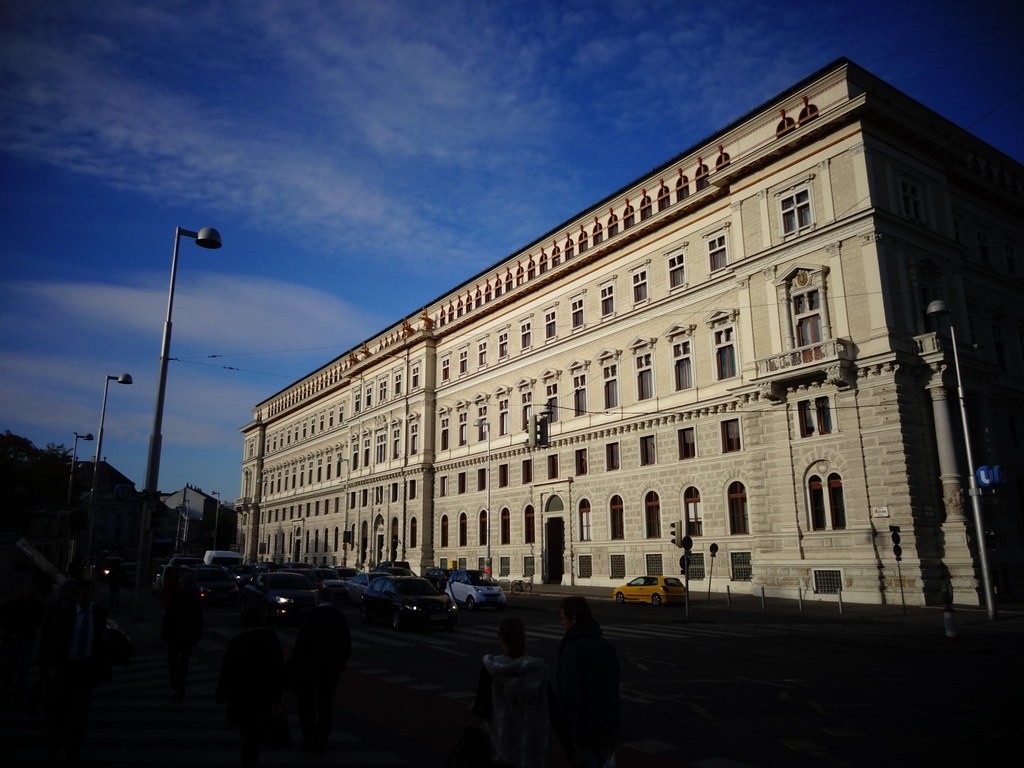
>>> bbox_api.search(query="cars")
[360,574,459,632]
[149,554,456,607]
[246,572,325,622]
[444,569,506,612]
[612,576,687,608]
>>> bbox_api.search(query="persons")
[159,573,203,698]
[0,563,115,768]
[214,599,285,768]
[468,617,552,768]
[546,597,623,768]
[289,578,352,761]
[105,558,123,609]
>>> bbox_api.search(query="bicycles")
[511,580,536,596]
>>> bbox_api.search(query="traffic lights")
[671,520,682,548]
[536,418,548,446]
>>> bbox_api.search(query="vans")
[202,550,242,567]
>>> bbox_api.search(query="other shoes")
[300,738,315,750]
[315,735,327,756]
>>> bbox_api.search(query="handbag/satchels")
[448,724,488,768]
[262,704,292,749]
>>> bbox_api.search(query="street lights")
[257,478,268,562]
[336,456,350,565]
[211,491,220,551]
[83,373,133,557]
[136,226,223,570]
[474,419,491,572]
[67,432,95,511]
[926,297,998,622]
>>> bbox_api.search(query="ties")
[79,610,89,650]
